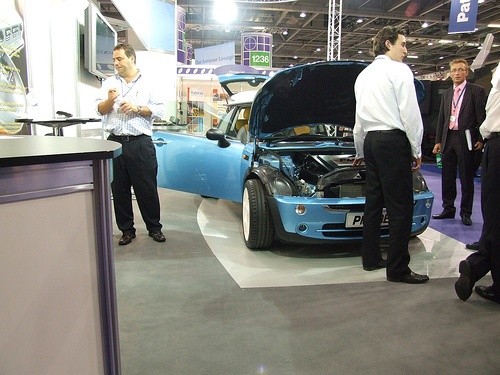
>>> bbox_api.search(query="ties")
[448,87,460,130]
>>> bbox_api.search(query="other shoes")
[465,242,479,250]
[462,215,472,226]
[455,260,476,302]
[475,284,500,304]
[431,211,455,219]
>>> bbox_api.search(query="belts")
[114,135,141,142]
[375,130,393,134]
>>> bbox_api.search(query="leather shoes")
[388,270,429,284]
[118,233,135,245]
[149,231,166,242]
[363,258,387,271]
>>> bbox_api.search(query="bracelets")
[136,105,140,114]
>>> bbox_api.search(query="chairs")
[235,119,248,130]
[237,108,250,144]
[289,126,315,136]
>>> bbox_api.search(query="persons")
[353,28,430,283]
[95,43,165,244]
[432,59,485,225]
[455,64,500,304]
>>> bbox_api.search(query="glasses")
[112,57,126,62]
[450,69,467,73]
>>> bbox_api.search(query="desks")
[0,135,123,375]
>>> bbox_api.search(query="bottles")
[112,91,131,116]
[436,150,442,168]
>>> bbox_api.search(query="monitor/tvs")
[84,3,117,81]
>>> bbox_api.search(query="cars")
[152,60,435,252]
